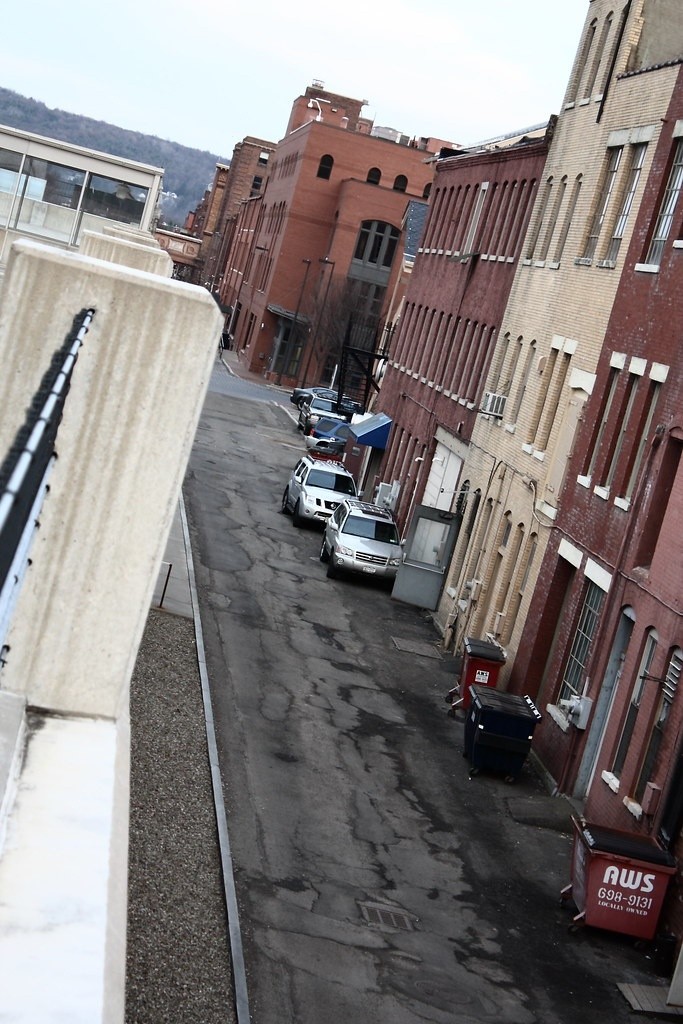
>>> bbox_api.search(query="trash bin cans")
[558,813,677,953]
[445,635,507,718]
[463,682,545,785]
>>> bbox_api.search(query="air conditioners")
[481,392,508,415]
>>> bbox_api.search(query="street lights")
[301,257,336,389]
[274,258,312,386]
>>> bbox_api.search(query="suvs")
[281,455,365,528]
[296,393,348,435]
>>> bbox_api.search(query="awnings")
[347,412,393,448]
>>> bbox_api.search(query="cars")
[308,440,347,461]
[319,500,407,579]
[304,418,350,446]
[289,386,360,411]
